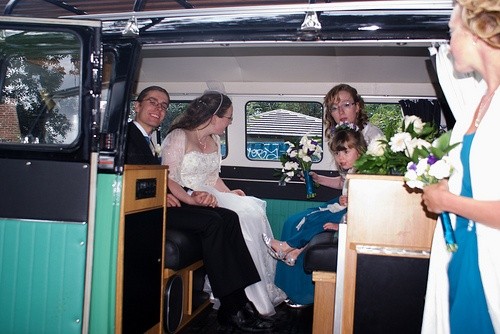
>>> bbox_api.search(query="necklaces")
[196,128,207,146]
[474,91,494,127]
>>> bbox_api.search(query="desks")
[341,174,448,334]
[115,164,169,334]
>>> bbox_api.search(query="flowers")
[353,115,463,253]
[273,133,324,199]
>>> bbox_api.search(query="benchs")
[165,228,211,334]
[304,231,338,334]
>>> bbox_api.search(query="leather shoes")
[217,308,273,332]
[243,302,273,321]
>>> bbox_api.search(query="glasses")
[140,99,170,111]
[218,114,233,122]
[329,101,357,113]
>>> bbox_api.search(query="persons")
[262,128,367,266]
[421,0,500,334]
[276,84,384,307]
[126,86,276,332]
[160,90,287,316]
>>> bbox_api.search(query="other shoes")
[267,245,297,267]
[262,232,286,261]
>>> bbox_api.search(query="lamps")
[300,11,322,31]
[122,16,142,35]
